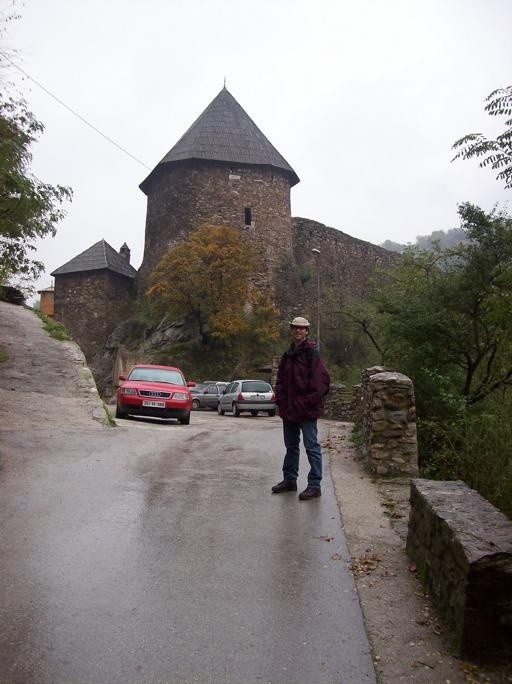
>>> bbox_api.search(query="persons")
[269,318,332,502]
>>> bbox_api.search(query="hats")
[289,316,310,327]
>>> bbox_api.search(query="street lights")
[312,248,320,356]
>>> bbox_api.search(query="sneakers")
[299,486,321,500]
[271,479,297,492]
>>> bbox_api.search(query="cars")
[116,365,196,424]
[189,380,277,417]
